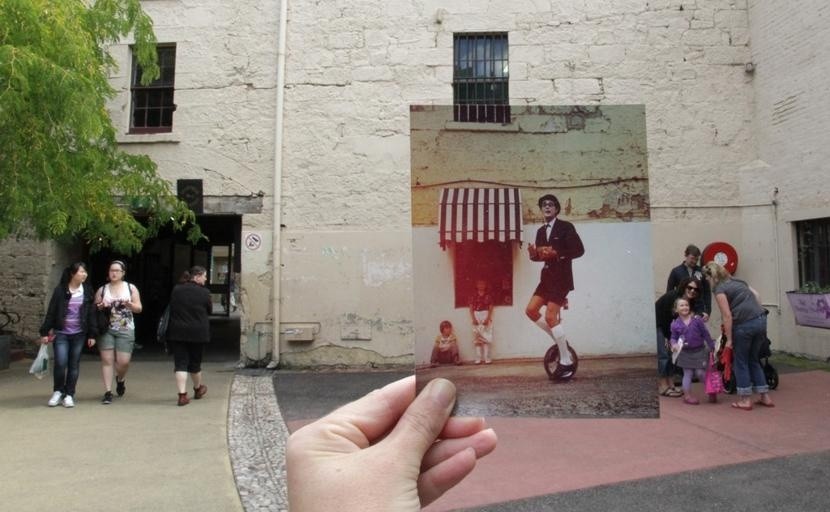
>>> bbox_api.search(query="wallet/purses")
[46,334,57,343]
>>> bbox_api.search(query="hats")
[539,194,561,214]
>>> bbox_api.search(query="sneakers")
[102,391,113,403]
[116,376,125,396]
[61,394,74,408]
[548,361,576,380]
[48,391,65,406]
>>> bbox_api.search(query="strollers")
[716,310,783,394]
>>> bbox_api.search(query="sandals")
[683,396,700,405]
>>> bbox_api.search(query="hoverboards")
[543,297,579,382]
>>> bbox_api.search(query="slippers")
[754,399,775,407]
[658,386,684,398]
[732,400,752,410]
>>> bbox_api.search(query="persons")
[669,297,715,405]
[430,320,462,367]
[470,280,493,364]
[286,375,498,512]
[701,262,774,411]
[168,266,212,406]
[221,271,238,312]
[38,261,99,408]
[656,278,700,397]
[525,195,584,379]
[666,245,711,386]
[94,259,144,404]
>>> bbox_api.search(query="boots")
[178,392,189,406]
[193,385,207,399]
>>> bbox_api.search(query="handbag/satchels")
[156,305,170,353]
[86,304,112,337]
[706,353,724,394]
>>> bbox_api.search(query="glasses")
[686,286,699,293]
